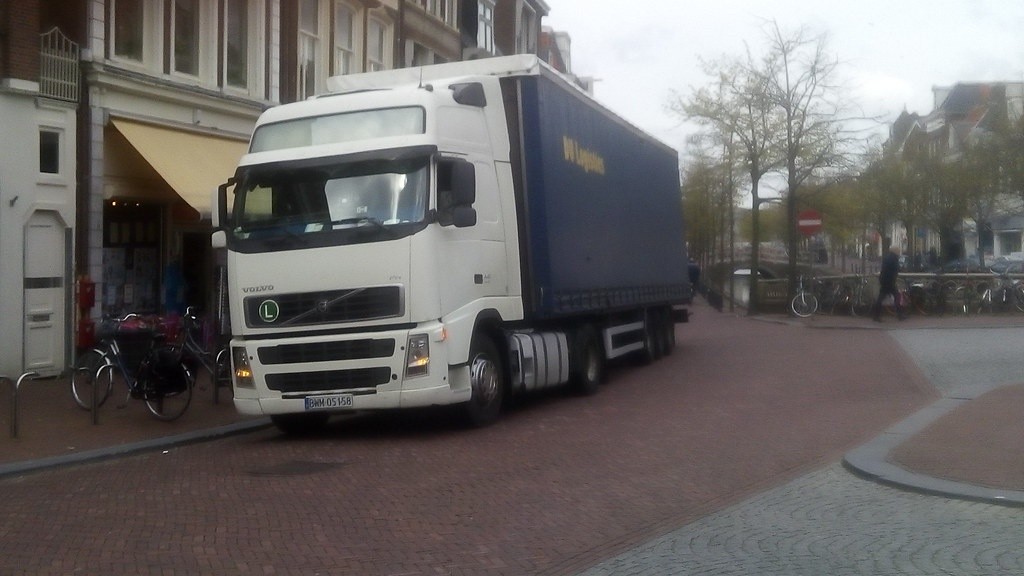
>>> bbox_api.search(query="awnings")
[110,117,272,221]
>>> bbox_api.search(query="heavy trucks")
[210,54,694,431]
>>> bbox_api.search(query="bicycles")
[791,252,1024,318]
[70,313,194,421]
[154,305,233,398]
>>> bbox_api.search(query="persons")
[687,258,700,285]
[873,246,909,322]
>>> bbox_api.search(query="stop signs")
[797,207,822,235]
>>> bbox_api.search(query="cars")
[988,255,1024,273]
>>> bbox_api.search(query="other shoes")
[897,314,909,320]
[873,315,885,322]
